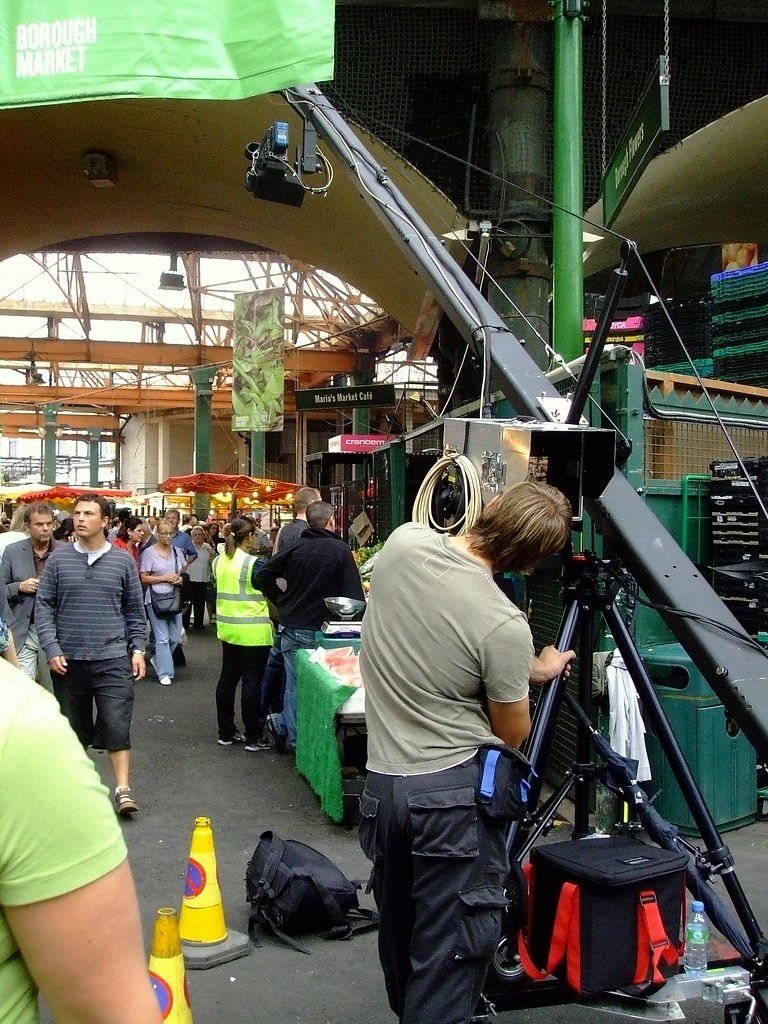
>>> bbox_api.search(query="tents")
[0,484,132,503]
[159,472,303,503]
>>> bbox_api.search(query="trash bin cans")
[638,641,758,838]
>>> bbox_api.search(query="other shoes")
[160,676,171,685]
[150,659,161,677]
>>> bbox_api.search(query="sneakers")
[244,739,271,751]
[218,730,246,745]
[113,786,139,814]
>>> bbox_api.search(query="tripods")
[481,552,768,1024]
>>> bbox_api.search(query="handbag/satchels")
[474,744,539,824]
[150,585,182,618]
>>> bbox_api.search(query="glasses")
[158,530,176,538]
[249,532,258,537]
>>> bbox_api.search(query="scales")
[320,596,366,638]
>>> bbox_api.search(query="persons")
[108,509,159,562]
[359,483,576,1024]
[258,501,367,753]
[34,494,147,813]
[0,656,161,1024]
[216,515,274,752]
[0,617,20,669]
[261,487,322,716]
[245,515,273,561]
[164,509,198,666]
[2,504,68,694]
[180,515,231,632]
[0,504,31,565]
[54,518,79,545]
[140,519,188,685]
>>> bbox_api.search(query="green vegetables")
[233,287,288,432]
[352,540,386,570]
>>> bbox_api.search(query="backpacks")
[244,831,380,954]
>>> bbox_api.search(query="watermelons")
[318,645,364,686]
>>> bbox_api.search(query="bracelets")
[257,550,259,553]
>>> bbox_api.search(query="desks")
[297,646,367,832]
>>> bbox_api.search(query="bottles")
[683,900,710,977]
